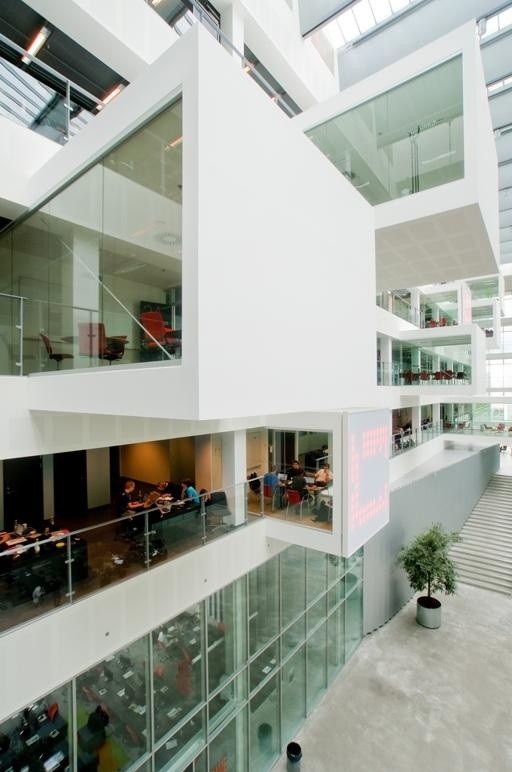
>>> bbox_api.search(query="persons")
[394,425,404,439]
[313,463,333,506]
[286,461,305,479]
[118,481,144,508]
[293,471,312,503]
[179,479,201,506]
[264,465,284,491]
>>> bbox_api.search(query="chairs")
[36,306,180,371]
[247,470,333,522]
[425,318,458,328]
[392,416,512,458]
[1,477,230,612]
[397,367,468,386]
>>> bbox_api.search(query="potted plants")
[395,521,466,629]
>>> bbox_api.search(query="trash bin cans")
[286,742,302,772]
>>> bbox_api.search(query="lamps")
[17,18,57,71]
[91,80,129,116]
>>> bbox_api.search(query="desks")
[0,606,286,771]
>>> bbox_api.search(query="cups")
[23,524,27,529]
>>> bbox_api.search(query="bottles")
[14,520,18,529]
[45,528,49,536]
[49,514,55,525]
[138,490,143,503]
[34,538,40,554]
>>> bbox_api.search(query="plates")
[5,537,27,546]
